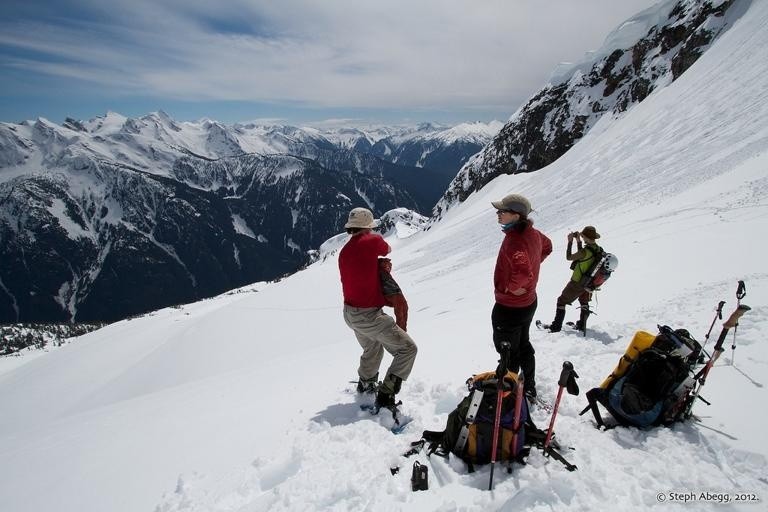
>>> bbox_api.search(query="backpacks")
[579,246,610,294]
[423,368,571,473]
[588,324,703,431]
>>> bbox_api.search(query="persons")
[544,225,606,337]
[491,194,552,397]
[338,206,418,424]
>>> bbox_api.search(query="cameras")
[572,233,577,237]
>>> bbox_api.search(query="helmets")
[604,254,618,272]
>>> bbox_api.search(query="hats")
[344,207,378,229]
[580,226,601,239]
[491,194,531,215]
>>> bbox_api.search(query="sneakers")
[357,372,381,393]
[535,320,562,332]
[565,322,586,336]
[374,390,403,425]
